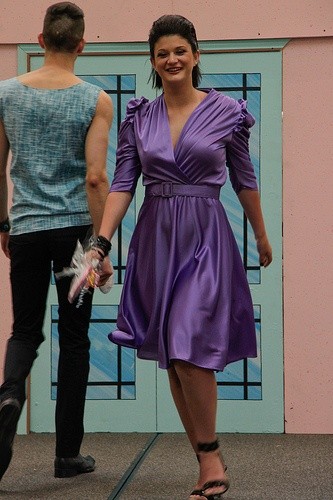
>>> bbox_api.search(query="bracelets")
[91,236,113,260]
[0,218,10,233]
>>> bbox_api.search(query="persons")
[82,12,274,500]
[0,1,115,478]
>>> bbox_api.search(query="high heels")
[198,434,230,498]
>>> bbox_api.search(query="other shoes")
[0,398,21,481]
[54,454,96,476]
[191,490,218,500]
